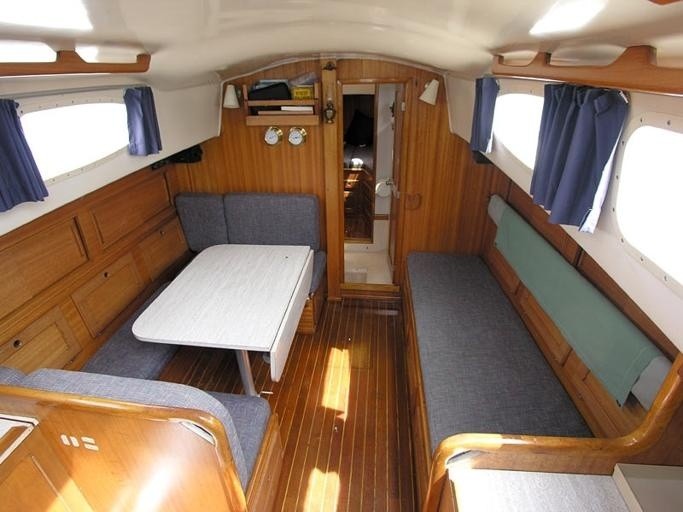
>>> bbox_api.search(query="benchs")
[1,256,284,512]
[401,250,596,511]
[176,190,329,332]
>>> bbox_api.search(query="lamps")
[223,84,242,110]
[418,78,440,106]
[323,96,337,124]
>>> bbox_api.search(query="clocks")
[264,127,306,146]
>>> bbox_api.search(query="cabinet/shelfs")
[342,171,378,227]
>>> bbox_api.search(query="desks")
[131,244,314,398]
